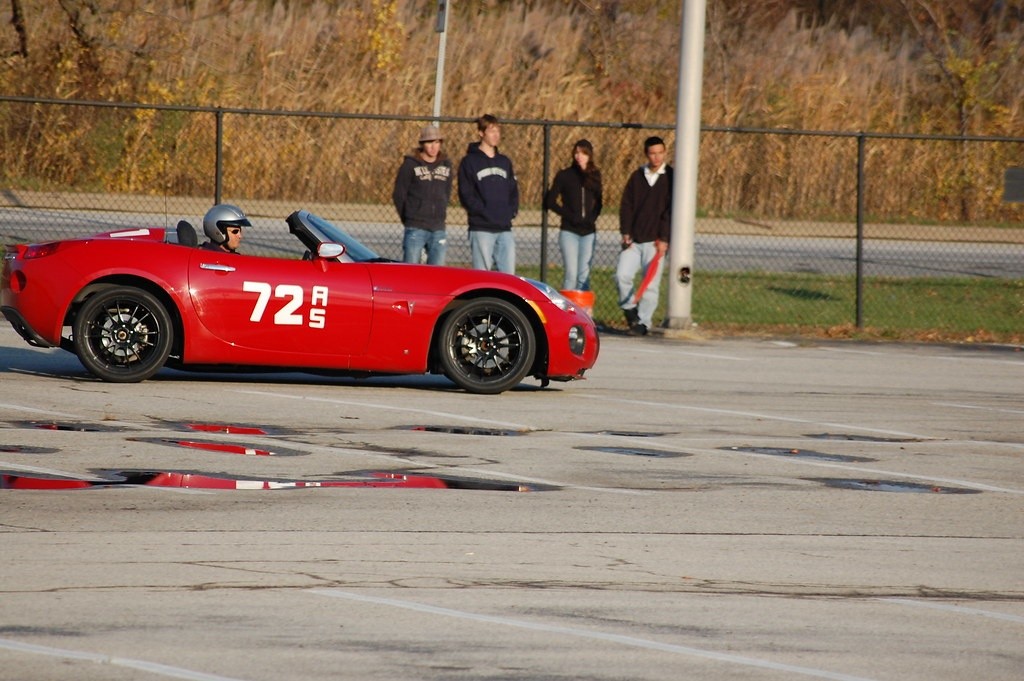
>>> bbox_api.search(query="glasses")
[227,229,242,234]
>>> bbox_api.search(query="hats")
[418,126,443,142]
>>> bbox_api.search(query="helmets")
[203,204,253,243]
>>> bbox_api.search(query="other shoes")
[624,308,641,328]
[627,324,647,336]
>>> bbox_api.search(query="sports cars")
[1,206,603,395]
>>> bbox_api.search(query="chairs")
[177,220,198,247]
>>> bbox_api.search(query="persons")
[392,125,454,266]
[457,113,520,274]
[196,203,253,256]
[614,136,675,336]
[546,139,604,291]
